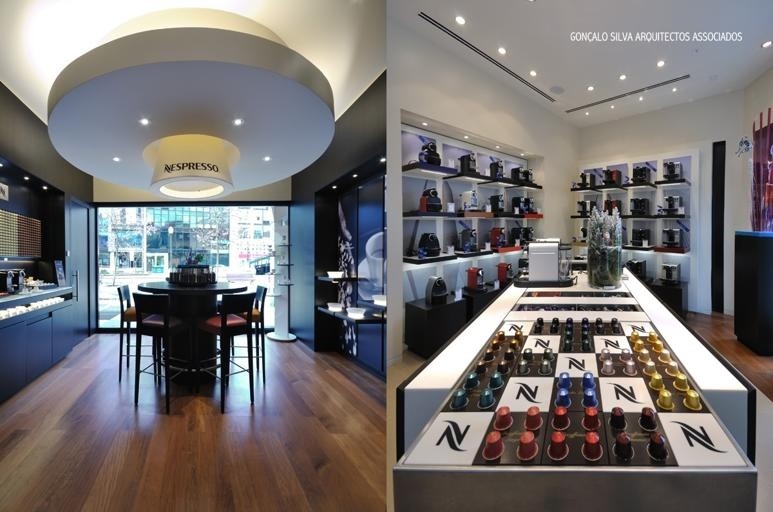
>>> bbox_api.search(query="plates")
[357,258,383,301]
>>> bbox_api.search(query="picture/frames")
[0,180,10,203]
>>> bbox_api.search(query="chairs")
[118,286,267,414]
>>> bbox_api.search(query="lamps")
[142,134,240,201]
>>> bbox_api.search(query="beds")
[138,282,248,384]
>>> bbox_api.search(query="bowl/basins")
[326,302,343,312]
[327,271,344,278]
[346,307,366,319]
[372,295,386,306]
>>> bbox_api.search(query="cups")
[365,231,383,288]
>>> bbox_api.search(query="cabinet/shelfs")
[734,231,773,356]
[571,156,692,321]
[401,124,544,360]
